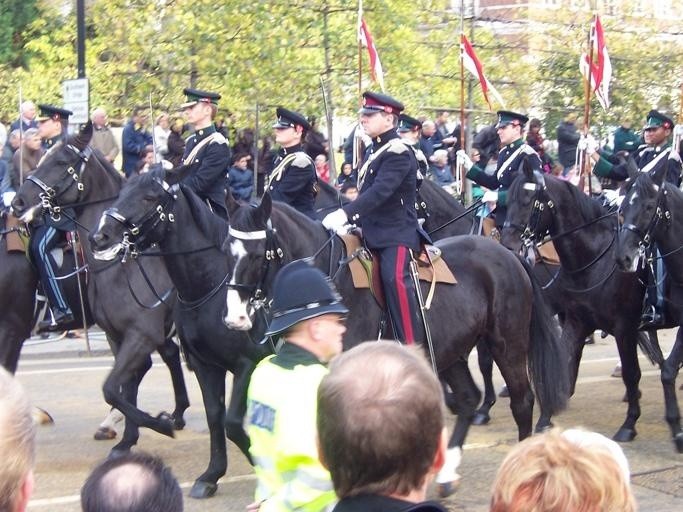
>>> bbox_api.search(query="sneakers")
[38,308,74,329]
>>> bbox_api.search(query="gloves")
[322,209,347,232]
[455,150,474,176]
[577,133,599,157]
[603,189,627,208]
[481,192,498,202]
[3,191,16,207]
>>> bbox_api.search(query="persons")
[0,89,682,511]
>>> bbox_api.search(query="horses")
[0,117,683,499]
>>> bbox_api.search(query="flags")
[459,35,503,109]
[357,8,388,93]
[593,14,615,107]
[578,45,607,111]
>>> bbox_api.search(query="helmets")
[263,259,350,336]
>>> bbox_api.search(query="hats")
[181,87,222,108]
[396,114,422,133]
[356,91,405,117]
[494,110,529,129]
[643,110,674,131]
[271,108,311,130]
[34,103,74,122]
